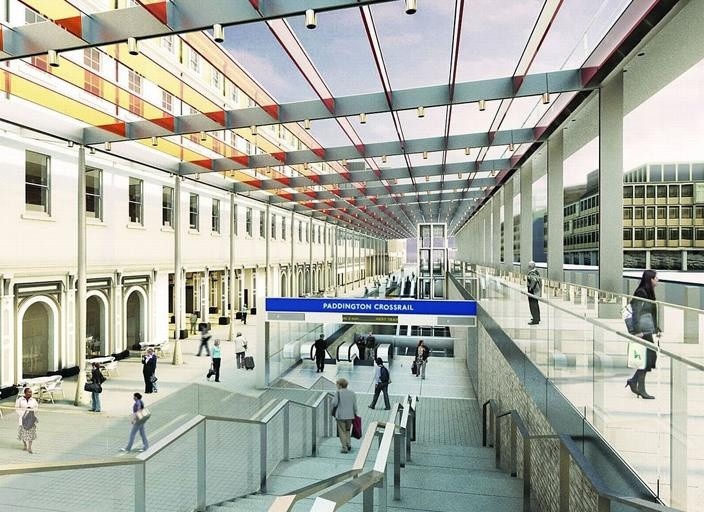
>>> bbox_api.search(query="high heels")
[623,379,656,400]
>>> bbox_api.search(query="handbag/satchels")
[83,369,106,394]
[21,410,37,429]
[411,365,417,374]
[331,405,338,417]
[350,415,363,439]
[621,303,632,320]
[624,313,655,336]
[625,340,646,370]
[132,408,151,424]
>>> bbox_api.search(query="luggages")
[243,356,254,370]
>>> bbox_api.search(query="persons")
[314,333,327,373]
[356,336,366,360]
[413,339,431,379]
[16,387,38,453]
[88,362,102,412]
[332,378,357,453]
[367,357,392,411]
[121,393,149,453]
[142,351,155,394]
[374,279,381,289]
[235,331,247,369]
[207,338,221,383]
[624,269,661,400]
[189,310,197,336]
[195,324,210,356]
[363,287,369,298]
[241,303,248,324]
[525,260,544,326]
[149,347,157,392]
[366,332,375,362]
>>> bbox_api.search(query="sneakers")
[88,408,100,412]
[22,447,33,455]
[120,447,145,453]
[527,318,541,325]
[340,445,351,454]
[367,403,390,410]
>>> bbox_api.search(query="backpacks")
[378,365,389,383]
